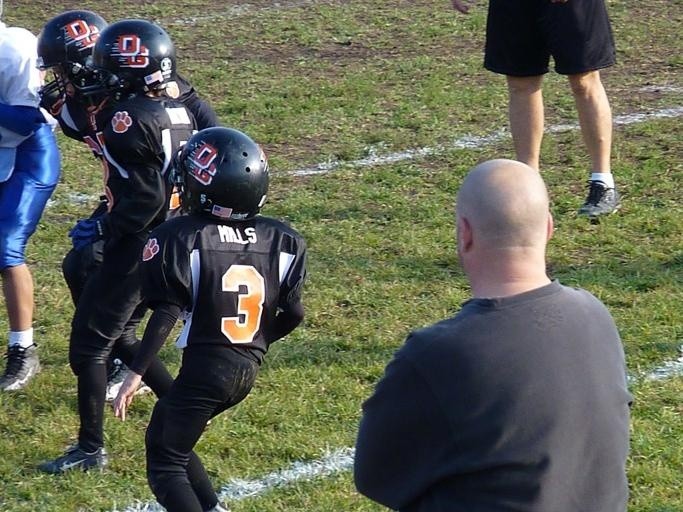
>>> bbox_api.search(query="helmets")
[34,9,176,93]
[174,127,271,222]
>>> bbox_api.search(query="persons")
[113,127,306,511]
[354,158,630,511]
[0,21,62,393]
[452,0,622,216]
[38,11,223,478]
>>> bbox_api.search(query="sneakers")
[39,447,108,475]
[103,370,152,402]
[577,184,623,221]
[0,347,41,391]
